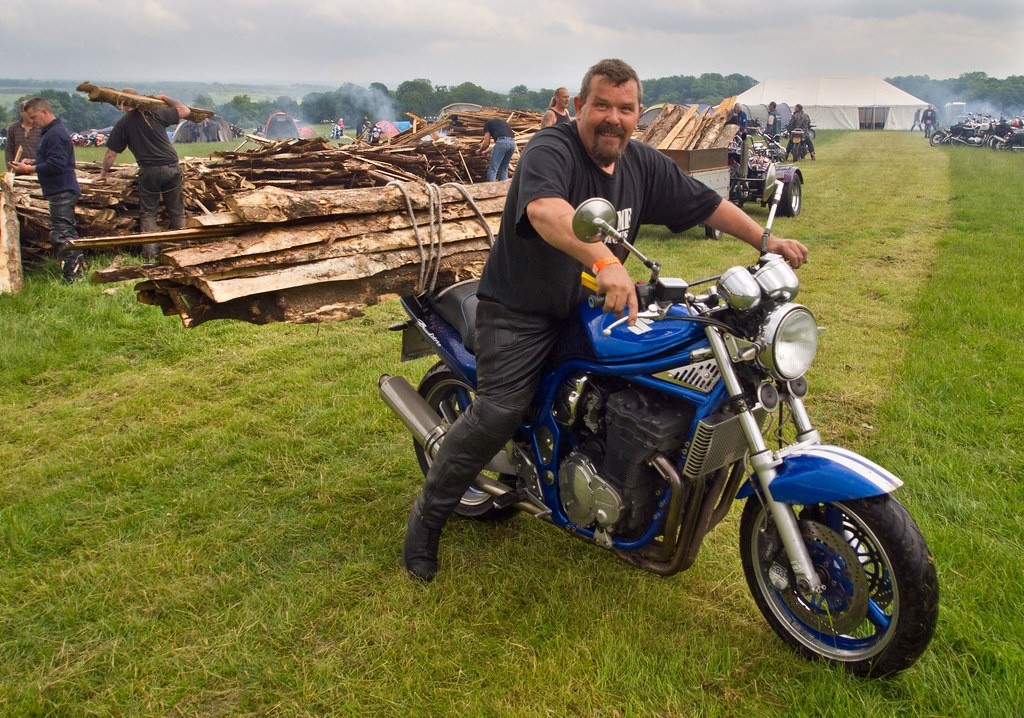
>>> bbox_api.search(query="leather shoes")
[404,509,443,584]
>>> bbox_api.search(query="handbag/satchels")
[926,120,932,124]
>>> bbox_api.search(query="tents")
[265,111,316,140]
[714,78,932,130]
[171,113,234,145]
[371,120,414,139]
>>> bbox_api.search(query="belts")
[498,136,514,138]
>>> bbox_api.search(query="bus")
[945,102,966,122]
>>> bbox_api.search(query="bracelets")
[24,165,30,174]
[592,255,621,275]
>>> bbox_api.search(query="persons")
[0,96,265,284]
[403,58,808,581]
[476,118,515,182]
[733,102,816,160]
[910,108,923,131]
[337,115,370,140]
[541,87,571,128]
[920,104,936,138]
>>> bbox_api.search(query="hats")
[796,104,803,110]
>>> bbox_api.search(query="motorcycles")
[791,128,807,162]
[230,125,245,137]
[745,118,765,136]
[782,121,817,139]
[71,132,110,147]
[365,126,383,144]
[332,124,341,139]
[929,112,1024,152]
[378,162,940,678]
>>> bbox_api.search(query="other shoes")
[810,152,815,160]
[60,253,86,285]
